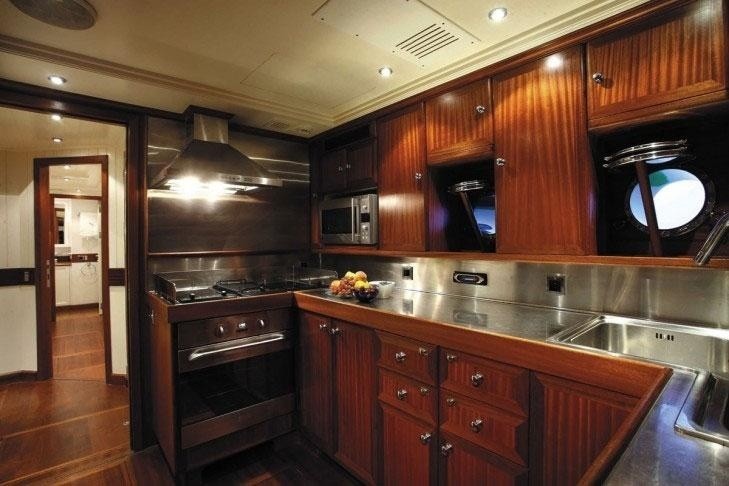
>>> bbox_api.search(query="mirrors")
[54,202,67,246]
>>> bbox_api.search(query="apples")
[345,271,355,280]
[330,280,339,288]
[354,271,372,288]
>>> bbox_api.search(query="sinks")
[543,314,729,447]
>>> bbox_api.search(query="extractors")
[147,141,284,196]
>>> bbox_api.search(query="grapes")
[333,276,354,296]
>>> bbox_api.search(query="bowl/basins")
[369,280,395,299]
[352,287,377,302]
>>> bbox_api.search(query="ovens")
[173,307,296,450]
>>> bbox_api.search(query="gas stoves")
[157,272,330,303]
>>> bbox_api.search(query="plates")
[323,289,355,299]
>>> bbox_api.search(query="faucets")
[692,213,729,266]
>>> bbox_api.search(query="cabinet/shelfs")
[425,77,493,164]
[377,102,450,252]
[70,261,99,308]
[55,263,70,308]
[314,121,377,193]
[492,43,605,255]
[378,330,530,486]
[298,307,377,485]
[531,371,641,486]
[586,0,728,130]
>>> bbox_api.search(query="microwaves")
[319,194,377,246]
